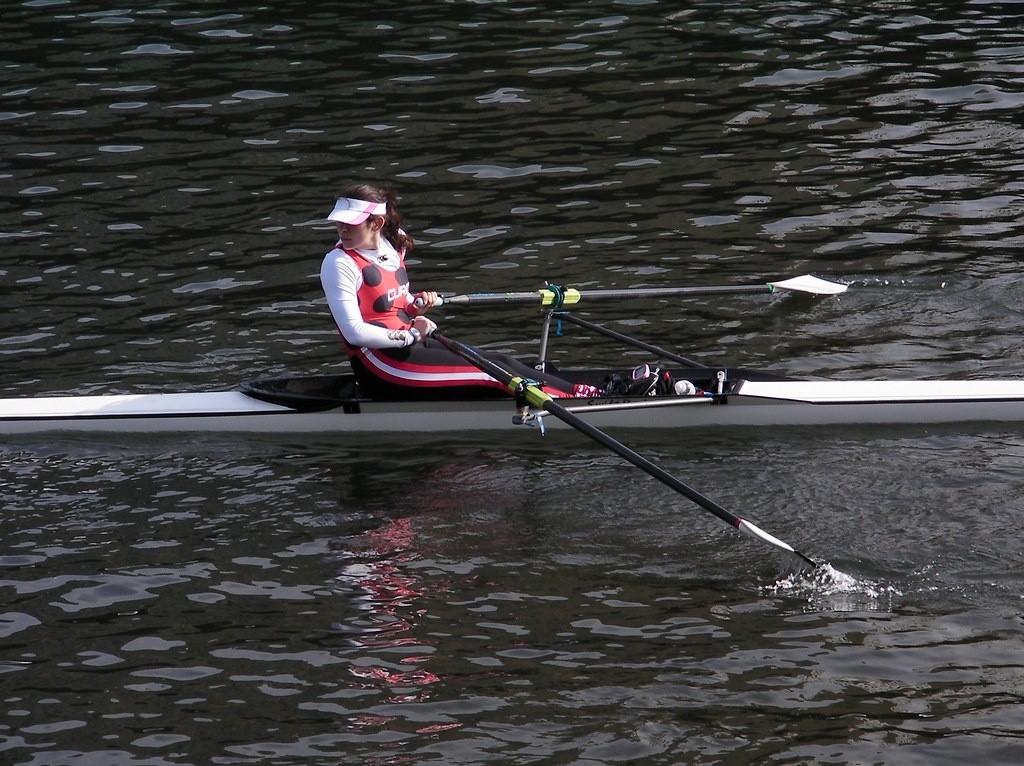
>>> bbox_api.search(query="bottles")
[674,379,696,395]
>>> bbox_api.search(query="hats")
[327,197,387,225]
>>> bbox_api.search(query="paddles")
[416,274,847,307]
[435,330,862,589]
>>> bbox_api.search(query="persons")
[320,184,669,401]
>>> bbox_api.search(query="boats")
[0,366,1024,436]
